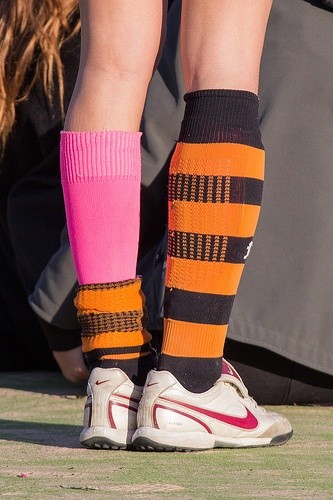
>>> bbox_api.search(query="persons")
[0,0,333,405]
[59,0,294,451]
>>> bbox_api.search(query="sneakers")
[133,357,293,451]
[80,367,143,449]
